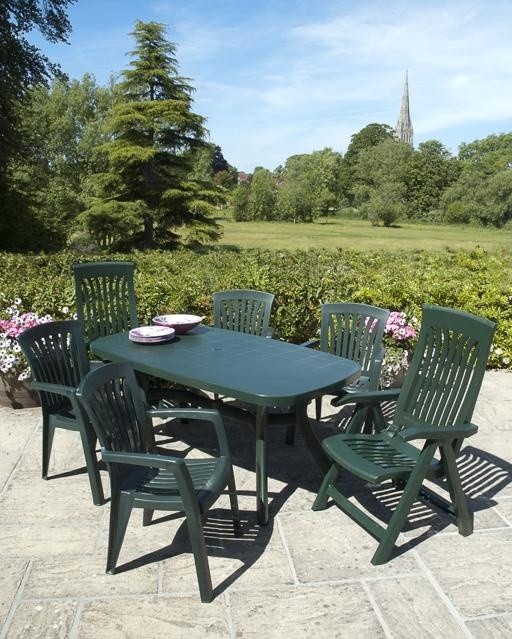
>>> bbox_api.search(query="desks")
[90,323,362,527]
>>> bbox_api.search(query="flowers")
[0,298,77,381]
[364,309,421,388]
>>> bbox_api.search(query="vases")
[4,374,40,408]
[391,364,405,388]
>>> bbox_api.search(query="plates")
[129,326,175,343]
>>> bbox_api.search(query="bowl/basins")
[153,313,202,331]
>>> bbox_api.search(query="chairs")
[314,303,497,564]
[72,261,168,420]
[16,319,146,506]
[76,359,243,603]
[285,303,390,444]
[214,289,274,400]
